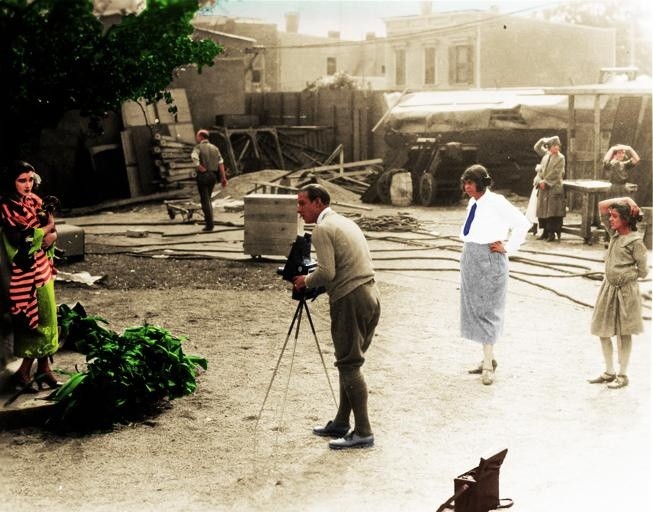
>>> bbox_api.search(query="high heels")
[33,373,64,389]
[12,372,39,393]
[528,227,555,242]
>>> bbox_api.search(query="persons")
[292,183,380,449]
[191,129,228,231]
[534,136,566,242]
[525,138,548,235]
[457,162,528,385]
[583,197,648,389]
[1,161,66,393]
[601,144,640,247]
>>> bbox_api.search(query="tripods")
[255,300,339,440]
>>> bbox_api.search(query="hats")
[548,136,562,146]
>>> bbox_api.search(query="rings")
[294,284,296,286]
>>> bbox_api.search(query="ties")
[463,202,477,235]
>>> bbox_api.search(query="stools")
[54,222,85,261]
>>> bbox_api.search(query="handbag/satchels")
[436,450,514,510]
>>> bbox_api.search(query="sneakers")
[328,431,374,449]
[312,420,350,438]
[480,369,493,386]
[469,359,495,372]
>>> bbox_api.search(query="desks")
[560,178,638,245]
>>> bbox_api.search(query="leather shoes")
[606,373,628,388]
[588,371,616,384]
